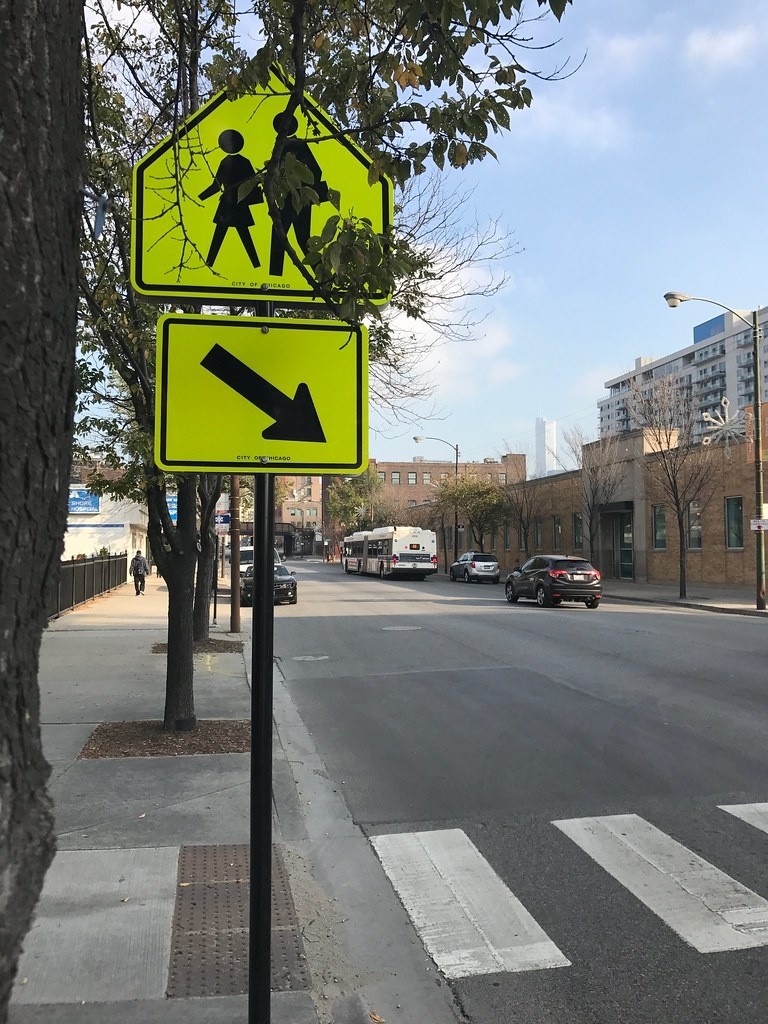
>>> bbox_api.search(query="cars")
[238,565,298,607]
[218,544,234,558]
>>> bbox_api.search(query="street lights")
[412,435,459,564]
[663,291,767,610]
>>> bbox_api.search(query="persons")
[130,550,149,596]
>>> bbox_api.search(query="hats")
[136,550,142,554]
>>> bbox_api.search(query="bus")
[340,526,438,581]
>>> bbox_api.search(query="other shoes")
[135,593,140,597]
[139,591,146,595]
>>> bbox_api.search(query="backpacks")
[132,556,145,575]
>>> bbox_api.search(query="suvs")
[229,546,282,577]
[449,550,501,585]
[506,553,603,609]
